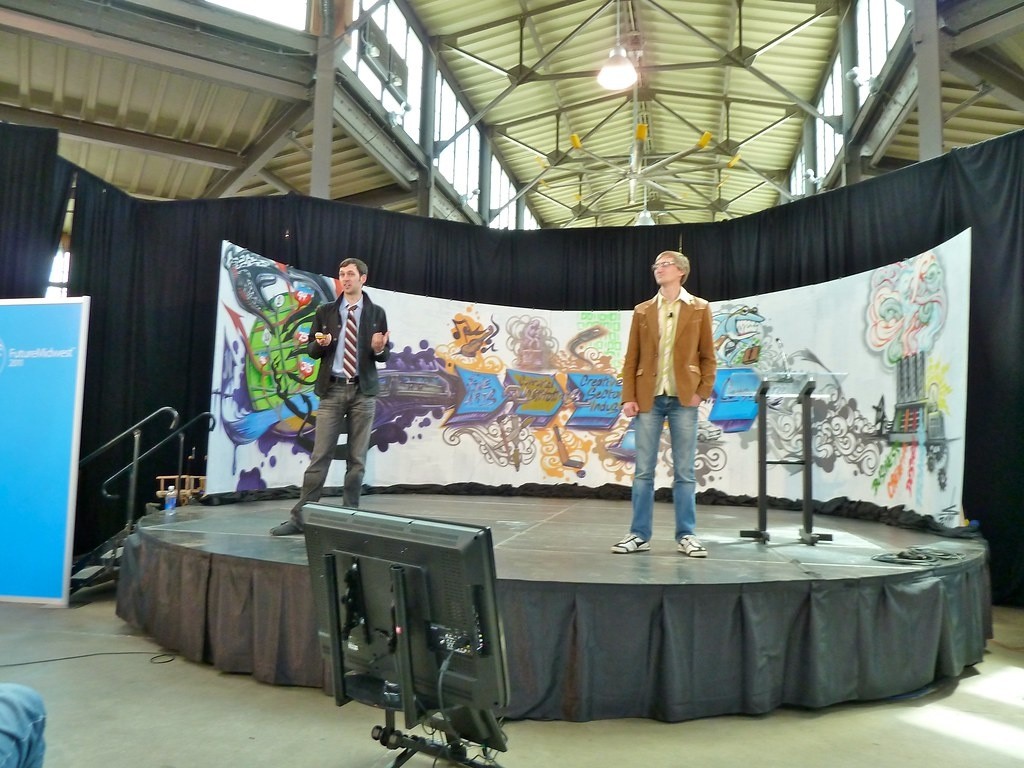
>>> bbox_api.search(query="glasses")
[651,262,676,270]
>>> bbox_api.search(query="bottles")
[165,485,176,515]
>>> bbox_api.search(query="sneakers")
[677,535,708,557]
[611,534,651,553]
[270,519,304,536]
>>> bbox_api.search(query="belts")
[330,376,359,384]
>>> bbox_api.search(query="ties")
[343,304,358,380]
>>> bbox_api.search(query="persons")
[0,683,47,768]
[270,258,390,536]
[611,250,718,556]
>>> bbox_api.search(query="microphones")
[670,312,673,317]
[776,338,783,347]
[348,314,350,319]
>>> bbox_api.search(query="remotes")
[316,335,328,339]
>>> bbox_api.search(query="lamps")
[846,67,870,88]
[392,76,402,86]
[804,168,823,184]
[467,188,480,201]
[596,0,638,90]
[396,100,412,119]
[635,185,656,225]
[367,43,380,58]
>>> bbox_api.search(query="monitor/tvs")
[302,499,513,709]
